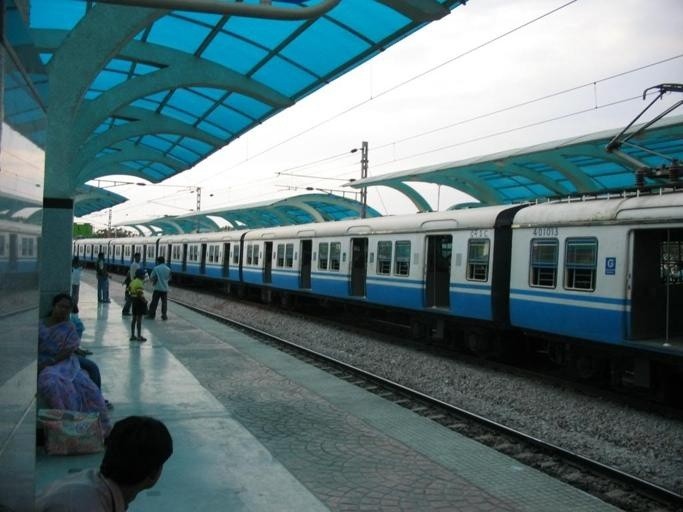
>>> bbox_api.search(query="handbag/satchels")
[152,275,158,285]
[37,409,105,456]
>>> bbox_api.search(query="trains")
[1,219,43,277]
[72,189,683,388]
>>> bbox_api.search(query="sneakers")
[162,317,167,320]
[129,336,137,340]
[136,336,147,341]
[144,315,154,320]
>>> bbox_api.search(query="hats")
[135,269,147,276]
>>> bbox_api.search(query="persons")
[121,252,148,315]
[125,269,147,342]
[71,255,86,306]
[34,413,173,512]
[71,295,113,412]
[37,290,110,452]
[143,256,173,320]
[120,270,129,300]
[94,251,112,304]
